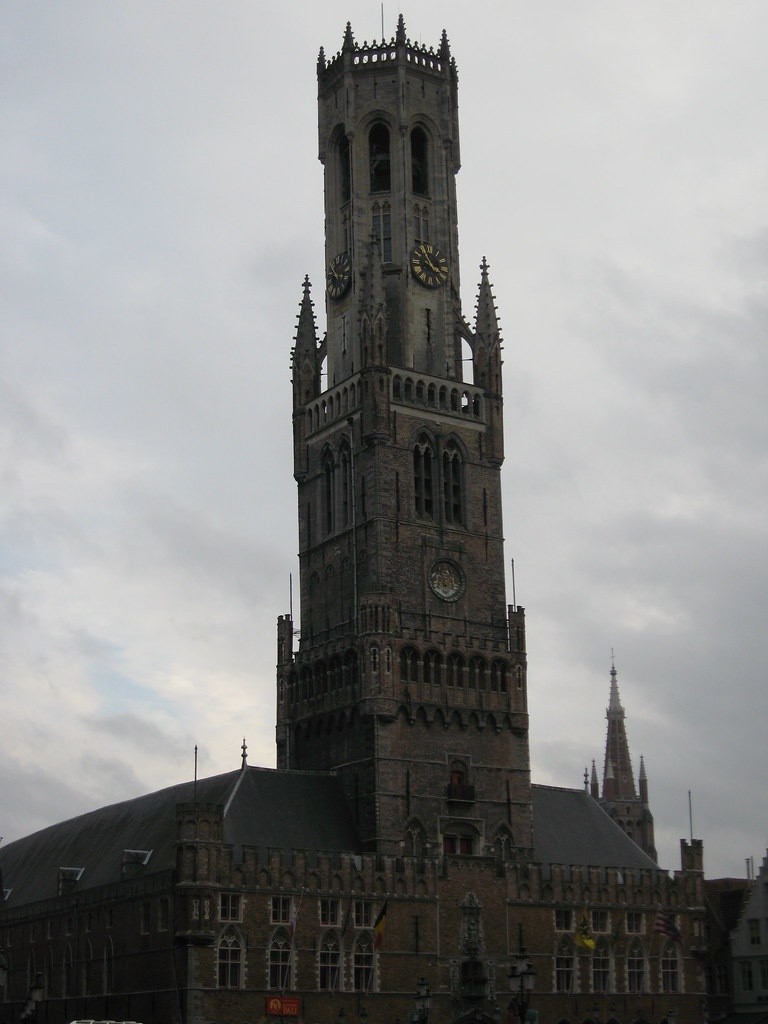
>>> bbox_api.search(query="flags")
[373,901,387,950]
[655,906,691,948]
[341,908,359,953]
[288,910,301,948]
[574,911,594,951]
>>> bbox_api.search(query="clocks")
[410,243,449,289]
[327,253,352,299]
